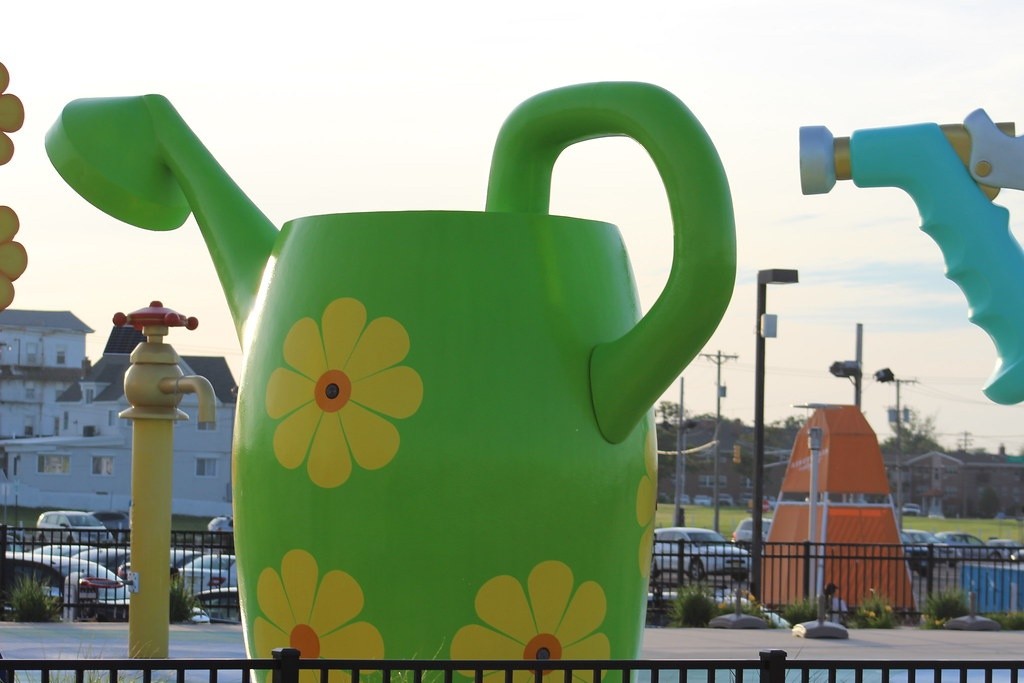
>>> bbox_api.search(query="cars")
[89,510,130,540]
[987,538,1024,562]
[33,511,118,544]
[652,527,753,584]
[903,528,961,563]
[903,503,920,515]
[732,517,773,549]
[935,530,1008,562]
[0,545,241,624]
[207,515,233,543]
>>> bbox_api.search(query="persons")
[825,583,848,623]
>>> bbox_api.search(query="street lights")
[747,266,800,604]
